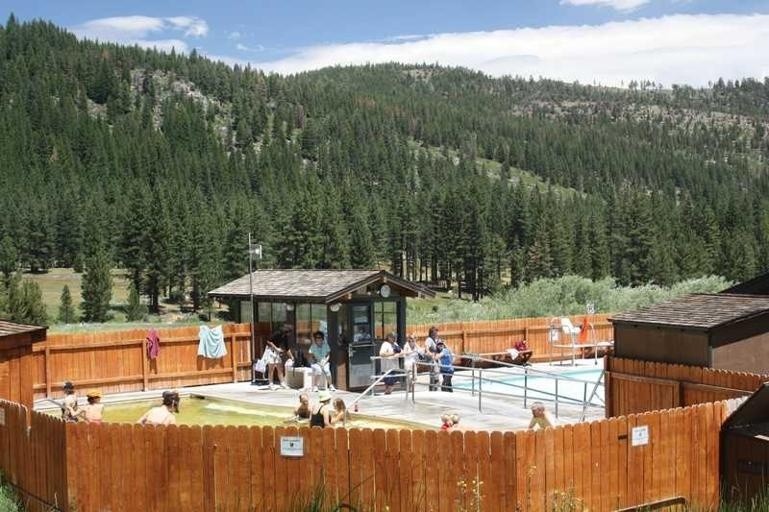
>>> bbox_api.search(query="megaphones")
[250,245,262,261]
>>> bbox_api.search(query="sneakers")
[269,382,289,390]
[313,387,318,392]
[329,384,336,391]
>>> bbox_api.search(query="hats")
[386,333,395,338]
[163,389,179,413]
[64,384,73,389]
[319,393,330,402]
[87,391,101,398]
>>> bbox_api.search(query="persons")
[528,404,551,429]
[61,381,78,421]
[332,398,352,425]
[300,331,337,392]
[295,395,313,422]
[67,390,104,423]
[136,390,181,425]
[263,322,295,392]
[439,414,451,432]
[309,393,334,427]
[378,325,454,393]
[448,413,464,433]
